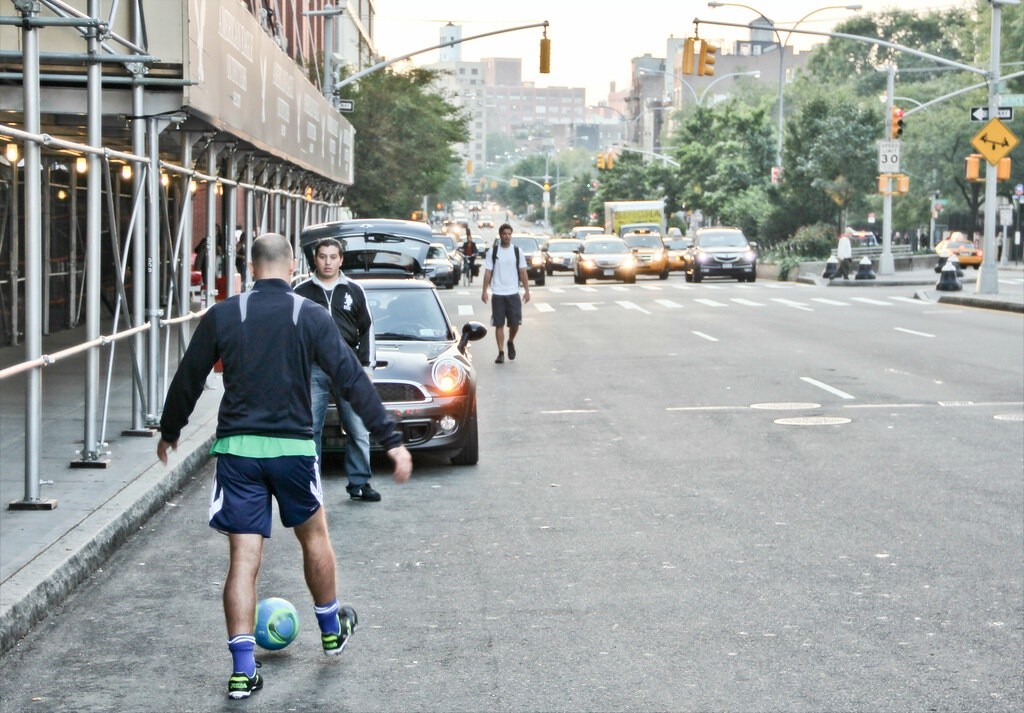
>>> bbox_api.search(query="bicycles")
[457,251,479,286]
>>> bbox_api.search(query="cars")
[535,234,551,251]
[571,234,636,285]
[542,238,584,276]
[661,236,693,271]
[852,230,878,247]
[293,214,487,466]
[935,231,982,270]
[413,243,455,289]
[622,229,669,279]
[683,225,756,284]
[428,200,514,248]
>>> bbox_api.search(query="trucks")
[603,200,666,239]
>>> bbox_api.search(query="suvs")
[571,226,605,242]
[428,233,463,279]
[458,235,490,259]
[492,232,545,287]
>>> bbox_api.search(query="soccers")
[251,597,301,652]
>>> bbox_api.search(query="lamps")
[305,187,314,201]
[3,142,225,196]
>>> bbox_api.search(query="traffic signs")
[970,106,1014,122]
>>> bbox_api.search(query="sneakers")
[323,604,358,656]
[346,482,381,501]
[228,667,263,700]
[507,340,517,360]
[495,353,504,363]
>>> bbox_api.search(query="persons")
[190,221,254,287]
[837,233,853,280]
[157,232,414,702]
[462,235,477,278]
[479,222,531,363]
[293,235,385,502]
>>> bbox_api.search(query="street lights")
[588,103,679,148]
[706,1,864,167]
[510,175,577,231]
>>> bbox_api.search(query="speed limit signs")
[878,141,899,172]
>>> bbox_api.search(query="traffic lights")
[412,210,423,221]
[891,105,904,138]
